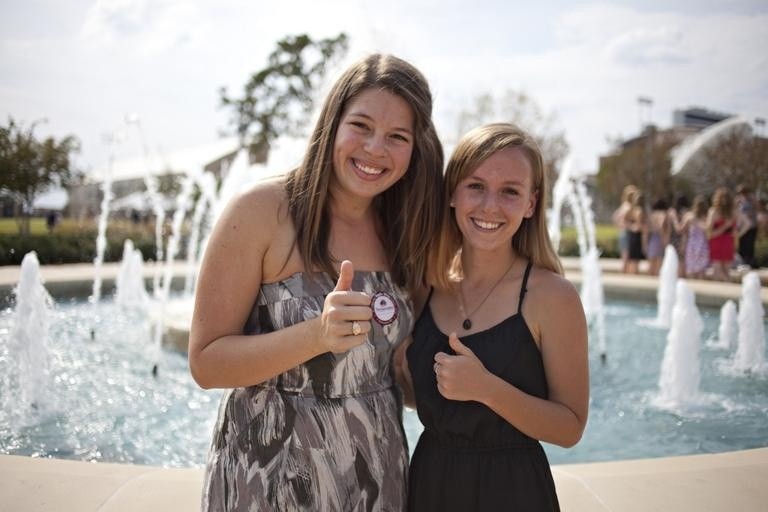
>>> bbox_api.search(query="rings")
[354,320,361,336]
[434,365,441,376]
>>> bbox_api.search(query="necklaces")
[459,253,519,331]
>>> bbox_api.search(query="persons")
[406,122,588,510]
[612,183,759,282]
[187,52,446,511]
